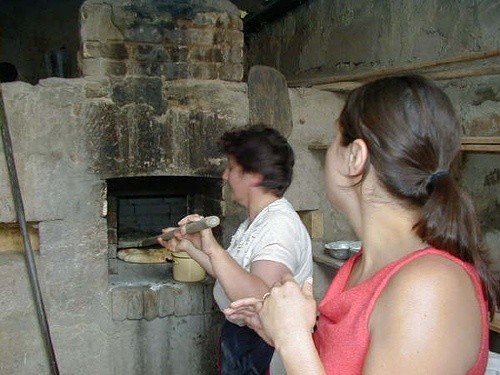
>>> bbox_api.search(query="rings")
[262,292,270,303]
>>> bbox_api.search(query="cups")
[51,52,71,78]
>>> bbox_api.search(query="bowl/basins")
[324,241,351,259]
[349,241,364,258]
[166,252,206,282]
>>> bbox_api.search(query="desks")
[313,254,500,333]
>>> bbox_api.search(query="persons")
[157,122,313,375]
[223,75,500,375]
